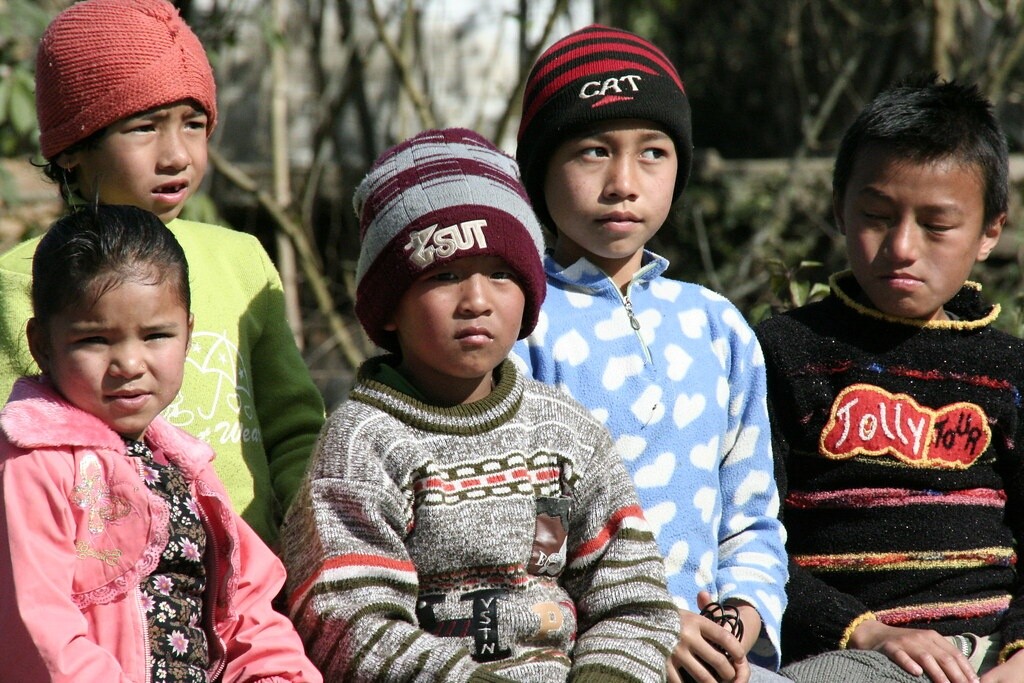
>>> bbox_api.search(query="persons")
[482,28,795,683]
[0,0,324,581]
[751,76,1024,683]
[0,201,327,683]
[261,130,685,681]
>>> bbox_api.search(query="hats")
[517,23,693,235]
[351,127,547,349]
[36,0,218,163]
